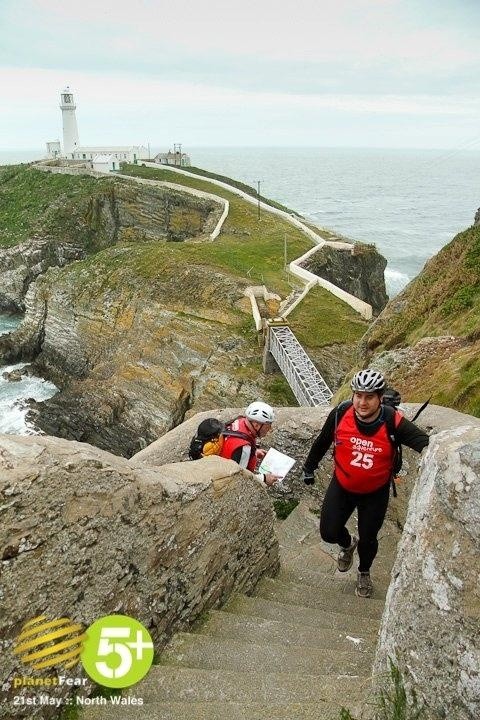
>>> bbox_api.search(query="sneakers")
[337,534,360,572]
[355,565,373,598]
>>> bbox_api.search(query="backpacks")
[188,415,256,461]
[334,388,402,498]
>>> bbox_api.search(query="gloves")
[303,471,315,485]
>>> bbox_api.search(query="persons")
[219,400,284,486]
[303,369,430,599]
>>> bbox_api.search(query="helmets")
[350,369,386,393]
[245,402,275,424]
